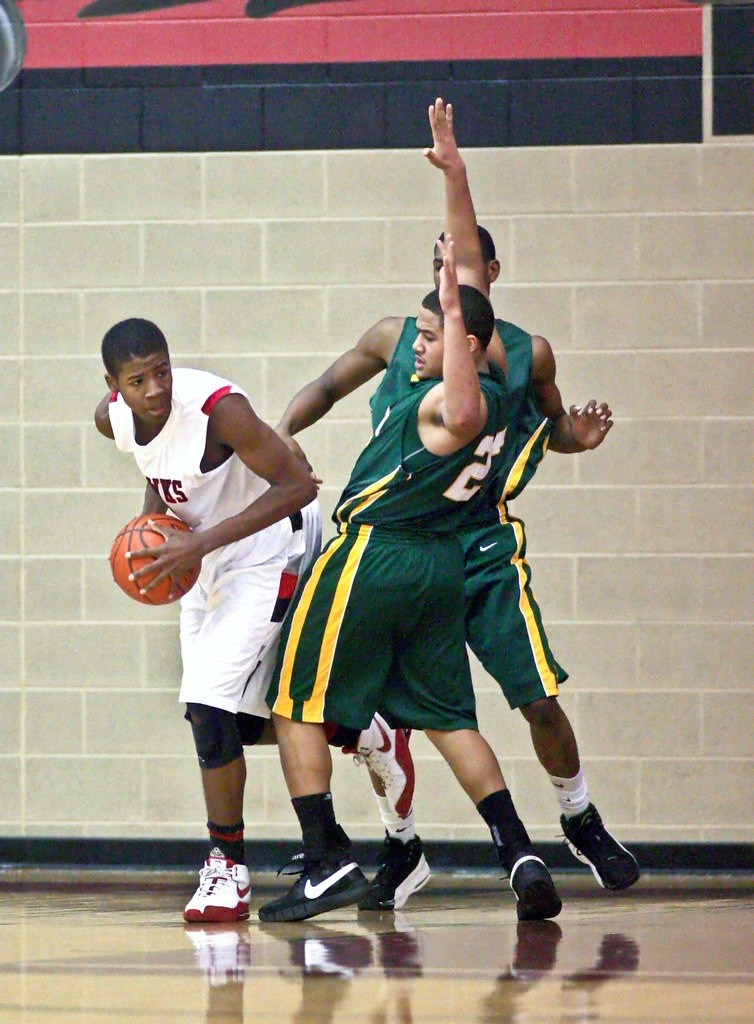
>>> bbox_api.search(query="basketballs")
[111,512,199,606]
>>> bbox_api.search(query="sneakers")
[182,845,250,921]
[288,931,373,974]
[572,934,638,985]
[555,801,639,890]
[496,853,562,920]
[377,927,424,977]
[358,829,439,909]
[259,856,369,923]
[181,921,250,986]
[511,919,563,982]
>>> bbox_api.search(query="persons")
[93,315,328,928]
[182,926,633,1023]
[257,97,564,926]
[273,222,642,913]
